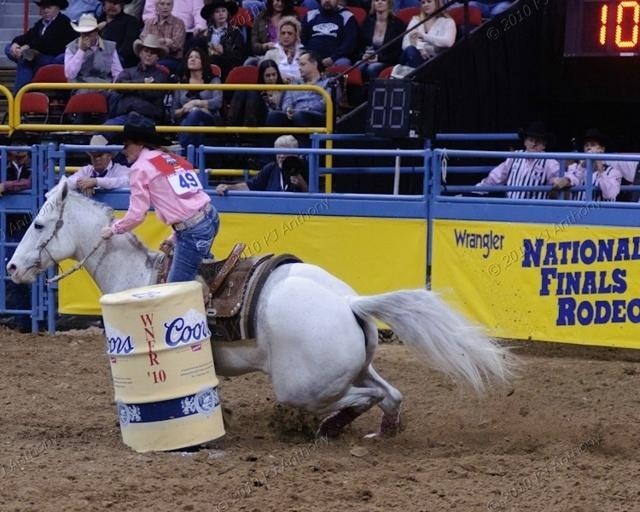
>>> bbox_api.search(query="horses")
[4,175,528,443]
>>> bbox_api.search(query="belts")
[173,204,210,231]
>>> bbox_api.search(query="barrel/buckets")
[100,281,227,452]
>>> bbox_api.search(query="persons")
[448,121,561,198]
[0,1,359,198]
[99,112,220,282]
[361,2,406,77]
[552,132,623,202]
[400,1,460,76]
[467,1,512,19]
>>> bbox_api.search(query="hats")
[87,119,172,154]
[282,155,309,182]
[201,0,238,19]
[71,13,107,33]
[133,35,169,58]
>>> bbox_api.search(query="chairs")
[0,0,483,212]
